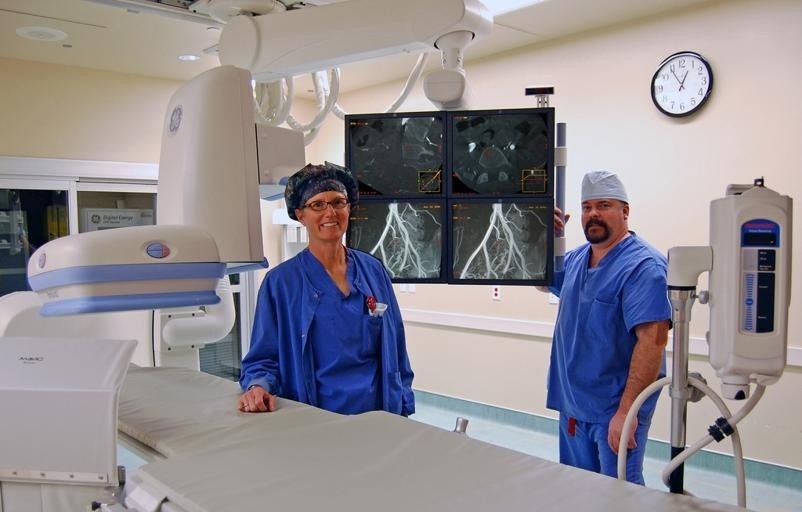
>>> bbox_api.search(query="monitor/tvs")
[344,106,555,287]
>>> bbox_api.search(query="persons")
[536,171,672,487]
[234,163,416,421]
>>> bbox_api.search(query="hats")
[581,170,629,204]
[285,161,359,221]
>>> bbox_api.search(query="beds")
[117,362,756,511]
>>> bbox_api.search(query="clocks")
[651,51,713,117]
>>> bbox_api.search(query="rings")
[242,402,249,409]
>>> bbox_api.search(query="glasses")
[303,196,351,211]
